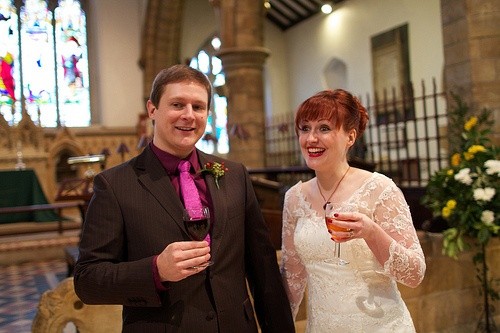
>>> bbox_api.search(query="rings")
[349,231,354,239]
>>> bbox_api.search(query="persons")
[279,87,426,333]
[73,64,296,333]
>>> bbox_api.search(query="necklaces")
[316,166,351,212]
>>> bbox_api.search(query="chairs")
[29,277,124,333]
[250,175,286,252]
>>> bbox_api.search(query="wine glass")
[324,203,359,267]
[182,206,214,268]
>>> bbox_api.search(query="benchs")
[0,199,86,234]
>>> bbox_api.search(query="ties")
[177,160,211,247]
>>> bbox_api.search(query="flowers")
[198,162,228,190]
[421,89,500,333]
